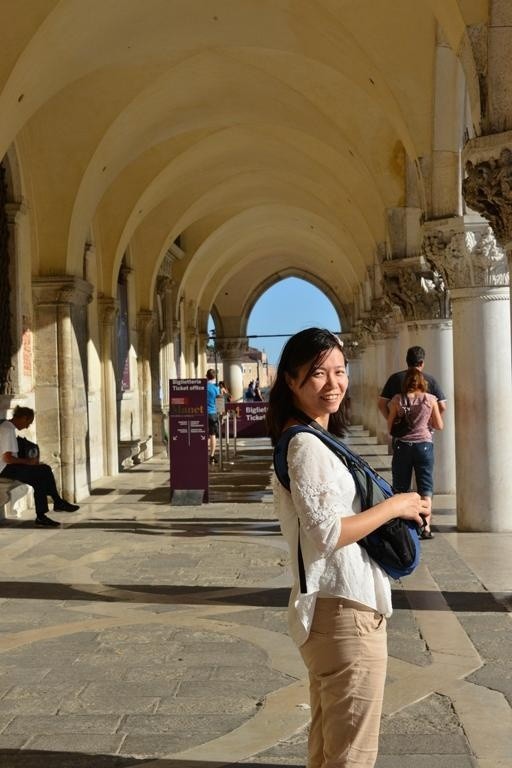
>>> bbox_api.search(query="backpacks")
[272,425,422,581]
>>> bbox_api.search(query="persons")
[206,369,228,463]
[246,378,264,401]
[378,346,448,539]
[0,406,79,526]
[266,328,431,768]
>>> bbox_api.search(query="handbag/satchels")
[16,435,38,463]
[389,393,414,438]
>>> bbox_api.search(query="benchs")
[118,433,151,474]
[0,476,34,523]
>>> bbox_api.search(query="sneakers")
[420,530,435,539]
[35,516,60,527]
[53,499,80,512]
[209,456,216,464]
